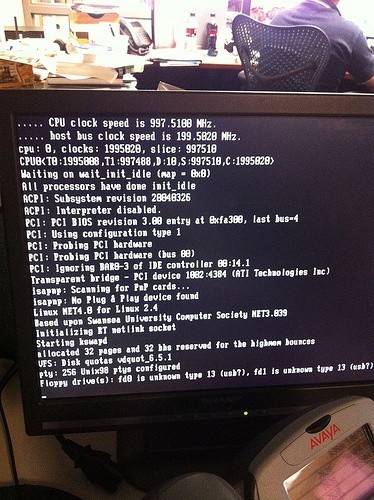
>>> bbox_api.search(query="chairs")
[232,14,332,92]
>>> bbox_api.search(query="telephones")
[120,20,152,49]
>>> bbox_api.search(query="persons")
[255,0,374,91]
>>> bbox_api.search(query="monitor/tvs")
[0,88,374,493]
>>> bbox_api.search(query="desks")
[140,49,354,81]
[0,360,243,500]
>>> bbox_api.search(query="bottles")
[206,13,218,57]
[184,12,198,49]
